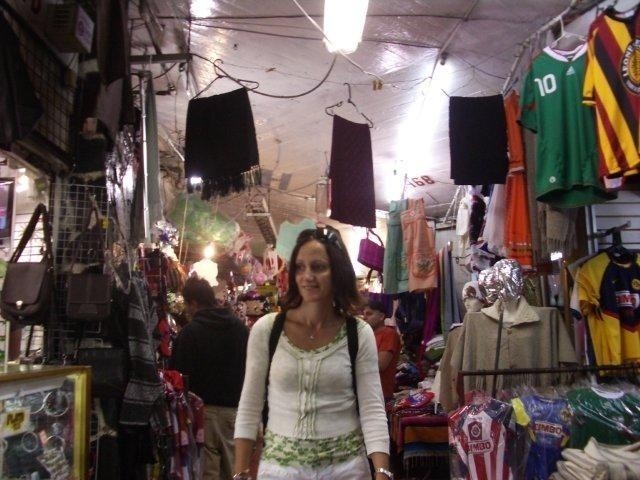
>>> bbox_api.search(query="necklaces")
[303,315,327,339]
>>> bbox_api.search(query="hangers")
[449,63,499,99]
[548,14,587,52]
[604,226,637,263]
[190,58,260,100]
[325,81,374,130]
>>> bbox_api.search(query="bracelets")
[233,469,250,480]
[375,468,394,480]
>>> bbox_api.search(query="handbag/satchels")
[359,238,384,270]
[67,273,112,319]
[1,262,51,324]
[73,347,126,398]
[395,294,425,335]
[355,270,393,317]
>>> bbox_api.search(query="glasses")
[297,228,342,250]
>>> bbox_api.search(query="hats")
[366,302,385,312]
[461,282,487,303]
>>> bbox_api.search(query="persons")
[233,228,390,479]
[432,258,579,407]
[168,277,250,480]
[362,300,401,404]
